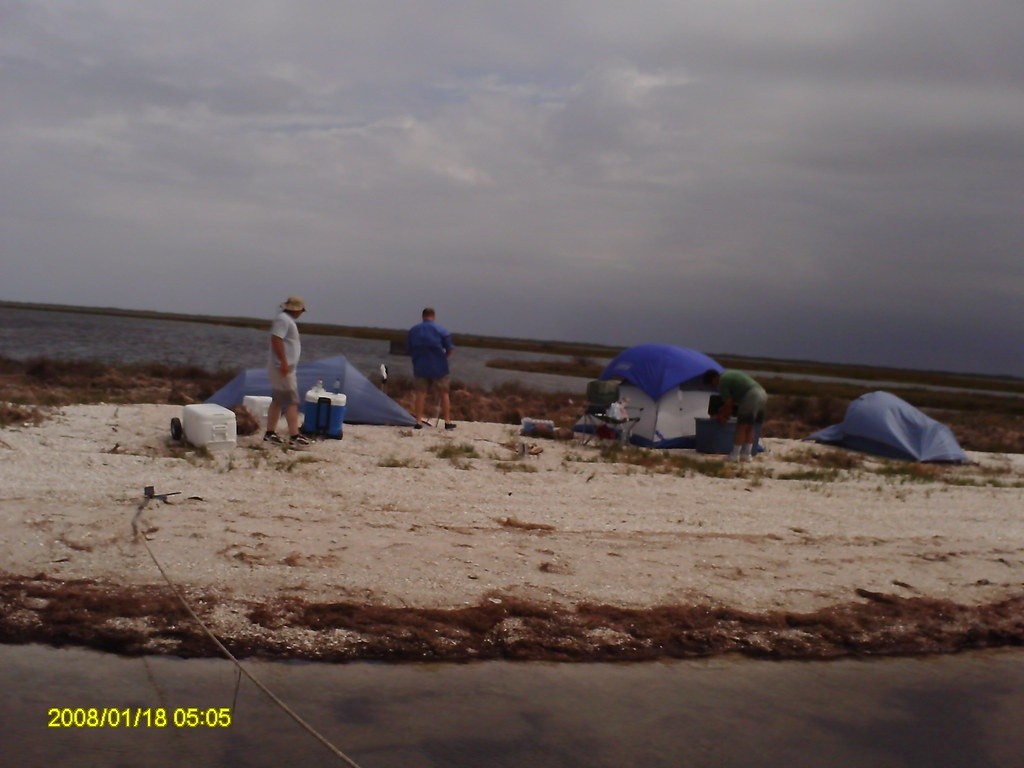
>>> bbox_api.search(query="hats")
[280,295,306,312]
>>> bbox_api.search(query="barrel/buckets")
[183,403,236,450]
[243,395,279,429]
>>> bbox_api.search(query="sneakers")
[263,431,284,443]
[289,434,316,445]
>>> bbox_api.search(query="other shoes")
[414,424,423,429]
[739,455,752,462]
[445,423,456,429]
[724,455,739,463]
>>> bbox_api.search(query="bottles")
[316,378,322,394]
[333,378,340,395]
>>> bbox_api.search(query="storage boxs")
[301,389,347,441]
[181,403,237,454]
[695,417,761,455]
[243,396,272,428]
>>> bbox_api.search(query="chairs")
[581,380,644,448]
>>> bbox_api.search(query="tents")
[803,388,973,467]
[594,341,727,402]
[204,353,417,425]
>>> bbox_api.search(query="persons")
[264,294,311,447]
[404,308,458,429]
[701,367,768,463]
